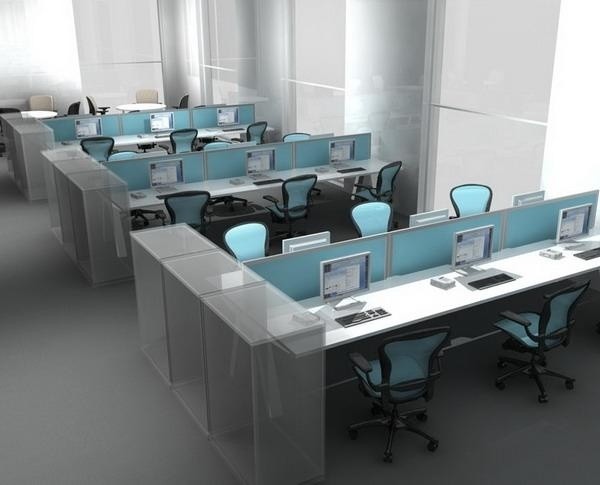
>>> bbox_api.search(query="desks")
[241,190,600,359]
[101,133,404,258]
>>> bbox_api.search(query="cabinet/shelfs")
[129,221,327,485]
[39,147,134,288]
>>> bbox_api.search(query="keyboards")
[335,307,392,328]
[466,273,516,290]
[222,128,245,132]
[336,167,366,173]
[155,135,169,138]
[573,247,600,260]
[156,195,170,199]
[253,178,284,185]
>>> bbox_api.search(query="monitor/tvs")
[556,203,593,247]
[150,112,174,132]
[512,190,544,208]
[245,148,276,179]
[329,138,356,169]
[282,231,330,254]
[74,118,103,139]
[452,225,494,276]
[149,158,184,195]
[318,251,372,311]
[409,208,449,229]
[217,107,239,125]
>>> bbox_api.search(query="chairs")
[222,220,270,261]
[347,326,453,464]
[350,200,393,237]
[450,184,492,217]
[350,161,401,202]
[492,278,590,403]
